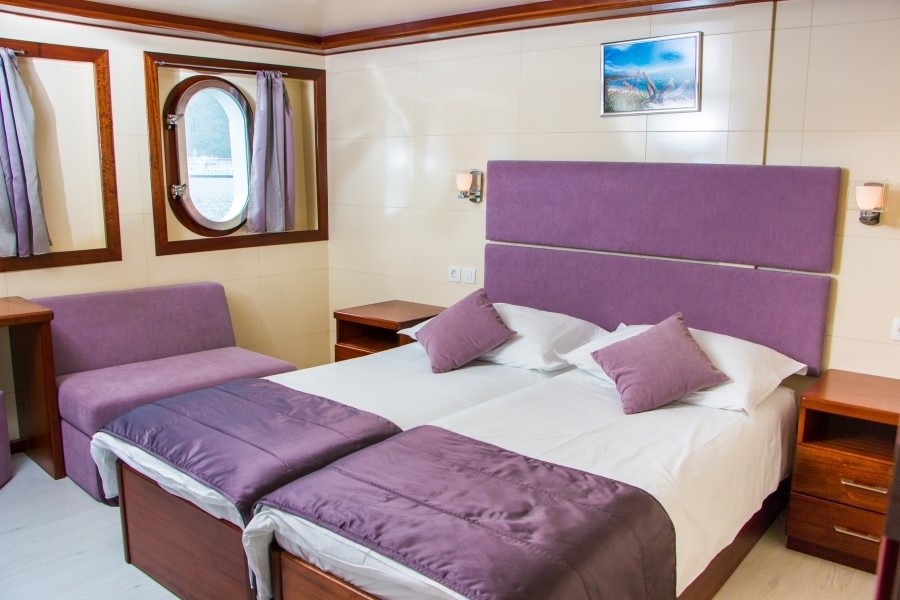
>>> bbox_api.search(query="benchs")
[29,282,297,506]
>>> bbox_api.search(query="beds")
[86,160,842,600]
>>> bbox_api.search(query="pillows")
[415,288,518,372]
[555,320,807,419]
[396,303,609,371]
[590,311,730,414]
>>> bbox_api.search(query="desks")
[0,296,65,480]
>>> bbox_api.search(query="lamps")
[856,182,885,226]
[456,168,483,204]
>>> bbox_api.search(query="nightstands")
[786,368,900,576]
[333,300,447,362]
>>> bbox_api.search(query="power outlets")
[447,266,461,282]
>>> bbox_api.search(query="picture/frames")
[600,31,702,117]
